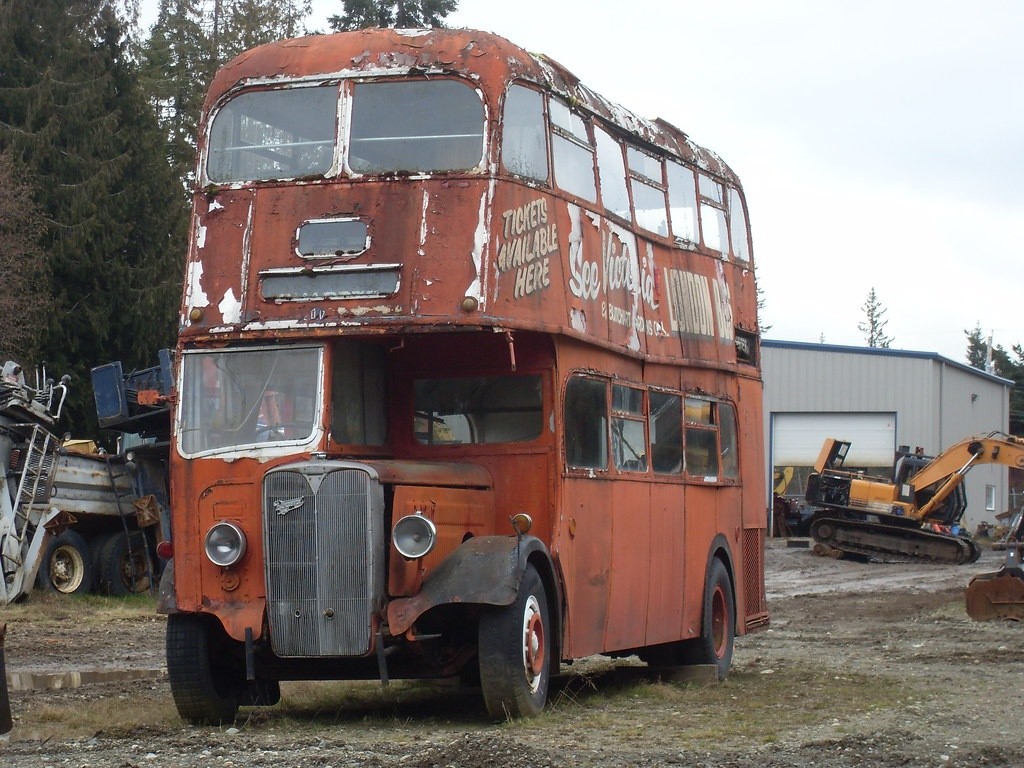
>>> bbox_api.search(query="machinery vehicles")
[991,506,1024,551]
[774,465,794,497]
[803,430,1024,629]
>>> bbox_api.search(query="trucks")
[0,345,176,600]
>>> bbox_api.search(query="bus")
[151,28,770,723]
